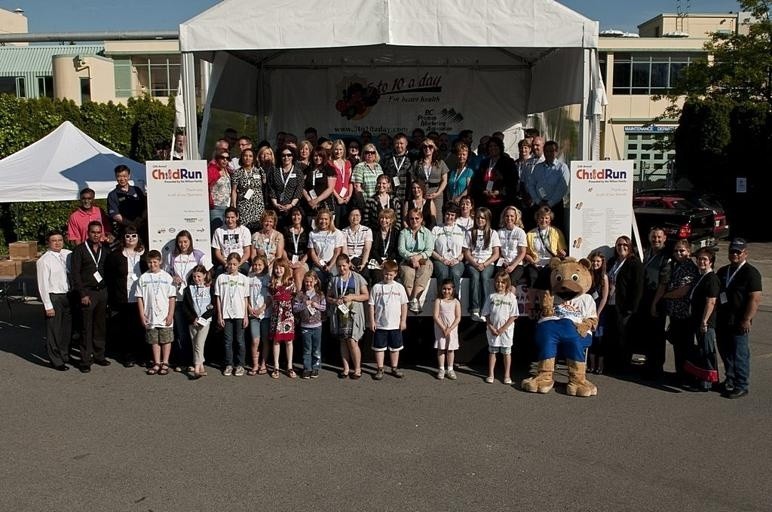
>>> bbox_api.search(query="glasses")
[617,243,628,246]
[281,153,292,157]
[219,156,230,160]
[125,233,138,239]
[365,151,376,154]
[424,144,434,150]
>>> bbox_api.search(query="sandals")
[123,359,207,377]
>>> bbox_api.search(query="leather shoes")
[340,368,362,379]
[50,358,111,372]
[721,379,748,398]
[486,376,512,384]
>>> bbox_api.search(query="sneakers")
[223,365,319,379]
[374,368,384,380]
[390,367,404,378]
[436,367,457,380]
[408,297,423,315]
[587,367,606,375]
[471,312,487,322]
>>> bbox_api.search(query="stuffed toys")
[520,256,598,399]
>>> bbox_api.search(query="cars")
[633,188,729,250]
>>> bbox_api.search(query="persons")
[107,164,147,227]
[35,229,73,370]
[68,188,113,245]
[70,221,117,372]
[666,239,699,385]
[714,237,762,399]
[105,225,150,368]
[687,246,721,391]
[133,127,665,386]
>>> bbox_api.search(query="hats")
[729,237,746,253]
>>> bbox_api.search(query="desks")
[0,279,44,322]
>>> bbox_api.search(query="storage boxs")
[0,260,22,281]
[9,241,38,261]
[22,259,39,281]
